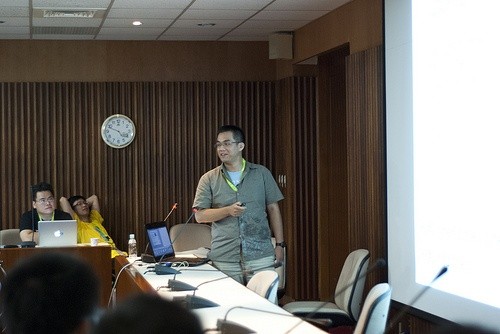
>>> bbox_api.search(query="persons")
[192,123,285,287]
[59,195,128,258]
[19,183,72,242]
[0,253,202,334]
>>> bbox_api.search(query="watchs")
[276,242,286,248]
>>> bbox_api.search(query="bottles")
[128,234,137,259]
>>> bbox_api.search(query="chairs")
[0,228,21,246]
[247,270,279,302]
[170,224,212,253]
[284,248,373,327]
[351,281,392,334]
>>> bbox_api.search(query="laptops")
[145,221,210,265]
[38,220,78,247]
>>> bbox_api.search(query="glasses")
[36,197,56,203]
[214,141,238,149]
[72,201,87,208]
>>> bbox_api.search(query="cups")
[90,238,98,246]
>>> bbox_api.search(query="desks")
[115,254,329,334]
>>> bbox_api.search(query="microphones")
[141,203,447,334]
[31,185,35,241]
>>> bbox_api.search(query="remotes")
[240,203,246,207]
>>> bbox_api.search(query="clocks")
[101,114,135,148]
